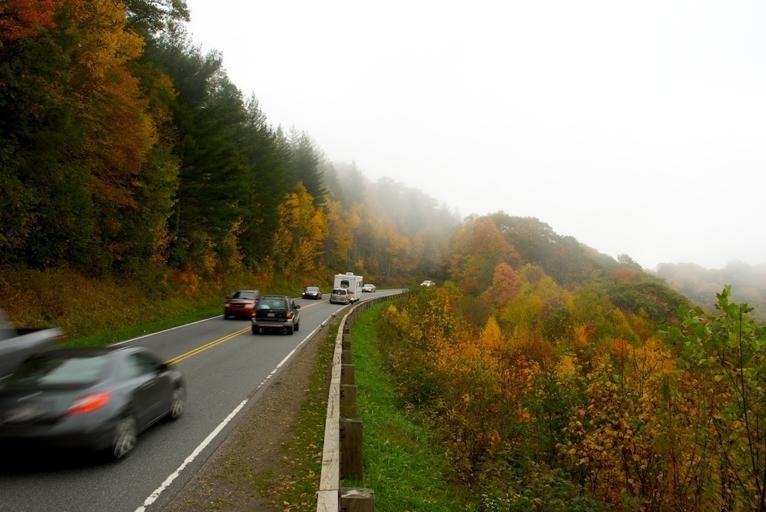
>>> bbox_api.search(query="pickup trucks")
[0,325,70,384]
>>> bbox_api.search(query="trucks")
[329,271,364,304]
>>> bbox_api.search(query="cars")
[362,284,377,294]
[302,287,322,300]
[4,343,187,477]
[251,295,301,335]
[420,281,436,288]
[223,290,259,320]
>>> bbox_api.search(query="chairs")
[262,301,273,307]
